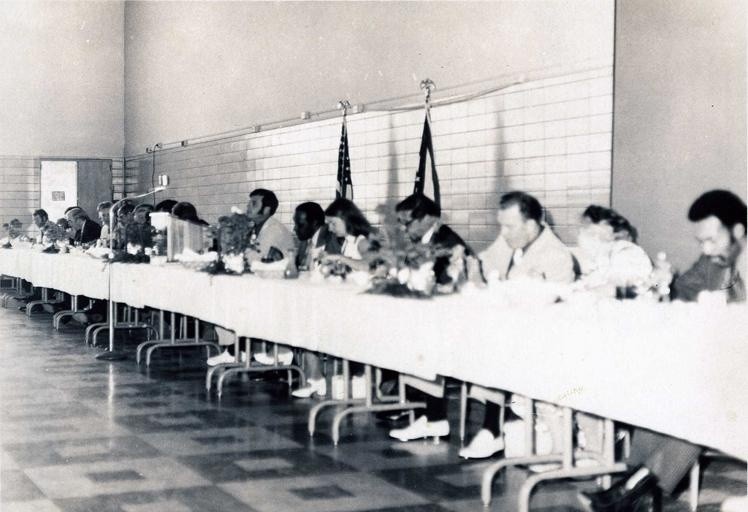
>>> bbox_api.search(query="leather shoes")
[205,349,246,367]
[390,415,450,442]
[457,430,506,459]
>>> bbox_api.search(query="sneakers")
[576,467,660,511]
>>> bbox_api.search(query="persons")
[264,201,334,383]
[574,186,748,512]
[370,193,470,421]
[252,197,380,400]
[507,204,658,483]
[386,187,577,462]
[1,189,295,366]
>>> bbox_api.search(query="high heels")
[253,349,293,366]
[293,376,326,399]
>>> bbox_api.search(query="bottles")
[648,249,674,307]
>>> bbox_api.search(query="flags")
[335,117,355,203]
[412,105,441,212]
